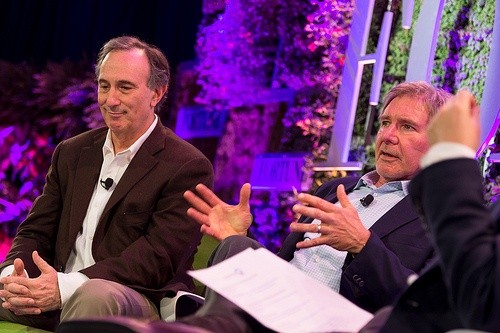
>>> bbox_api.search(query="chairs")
[0,230,222,333]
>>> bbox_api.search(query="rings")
[318,224,321,233]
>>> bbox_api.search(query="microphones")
[360,194,374,207]
[101,178,113,190]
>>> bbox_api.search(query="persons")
[183,81,456,333]
[0,36,214,333]
[358,86,500,333]
